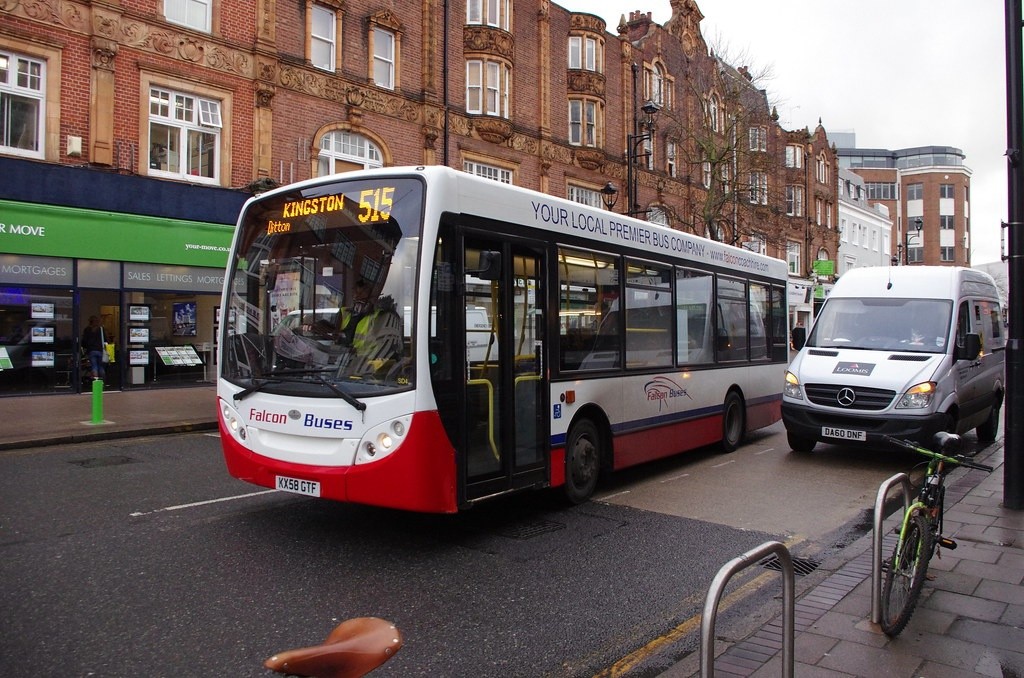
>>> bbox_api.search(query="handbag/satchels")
[102,349,110,363]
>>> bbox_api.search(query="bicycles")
[882,432,994,637]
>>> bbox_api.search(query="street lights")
[905,217,924,265]
[897,242,903,262]
[601,101,660,217]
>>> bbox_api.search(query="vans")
[780,264,1005,452]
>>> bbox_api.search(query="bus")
[215,166,796,515]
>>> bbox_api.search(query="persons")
[588,301,613,335]
[81,315,108,392]
[292,271,404,387]
[7,325,24,342]
